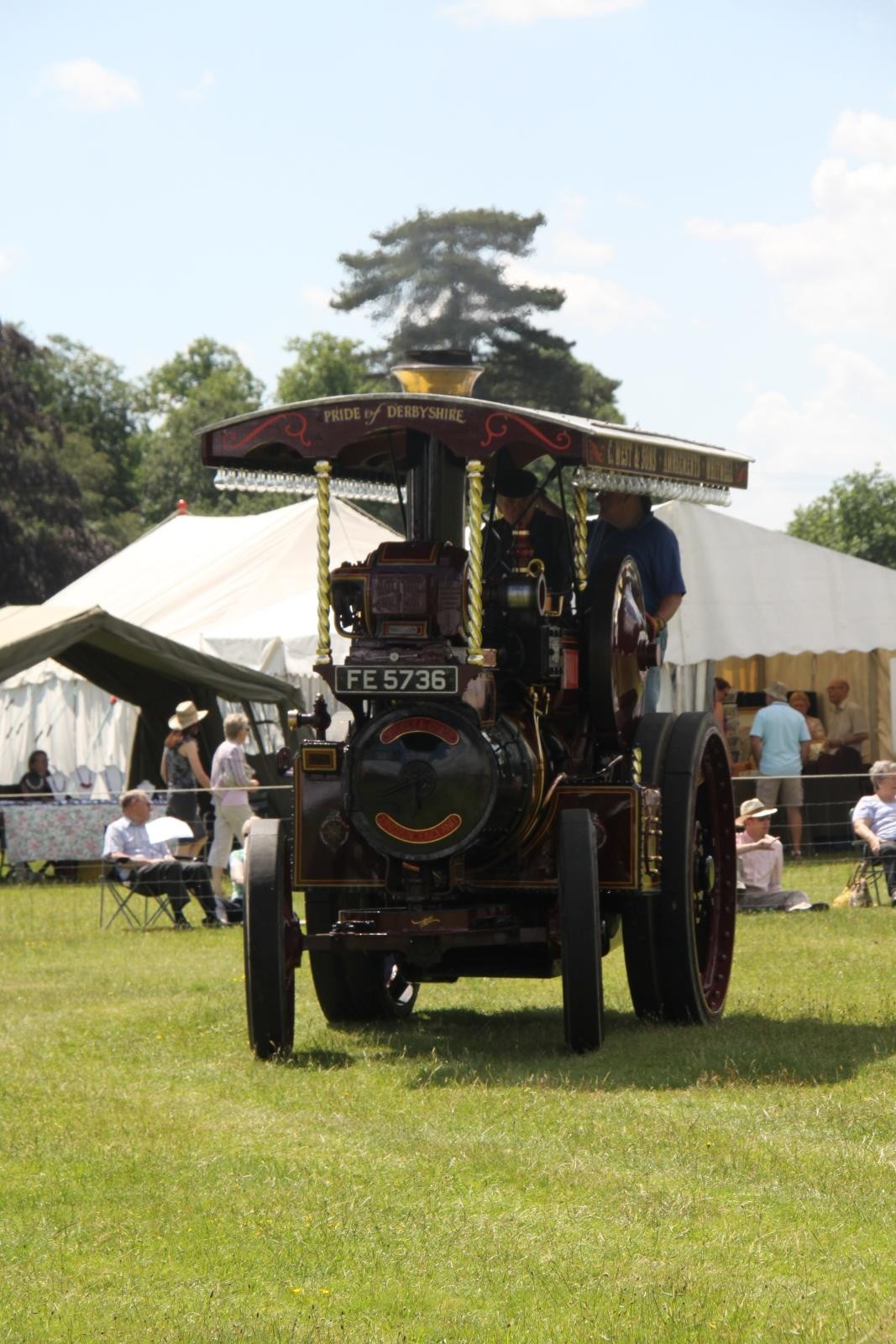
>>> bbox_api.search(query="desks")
[734,764,874,843]
[0,800,201,882]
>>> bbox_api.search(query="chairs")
[849,808,896,906]
[98,825,176,930]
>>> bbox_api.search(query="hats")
[167,700,209,731]
[762,681,788,701]
[734,798,778,826]
[494,468,538,498]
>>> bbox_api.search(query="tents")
[0,602,306,877]
[586,494,894,775]
[0,489,410,794]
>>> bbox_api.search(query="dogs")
[833,877,874,908]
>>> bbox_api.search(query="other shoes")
[891,890,896,905]
[788,902,830,914]
[790,853,802,861]
[202,916,230,928]
[176,921,191,930]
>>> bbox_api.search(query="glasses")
[243,727,250,731]
[749,815,772,821]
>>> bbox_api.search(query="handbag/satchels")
[832,878,873,908]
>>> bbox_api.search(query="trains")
[188,343,756,1061]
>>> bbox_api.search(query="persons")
[747,681,810,865]
[479,468,576,620]
[207,713,261,896]
[229,816,261,912]
[20,750,79,884]
[820,679,869,773]
[100,790,225,930]
[789,691,823,778]
[711,674,732,771]
[852,760,896,909]
[159,700,211,860]
[734,797,830,914]
[538,486,686,711]
